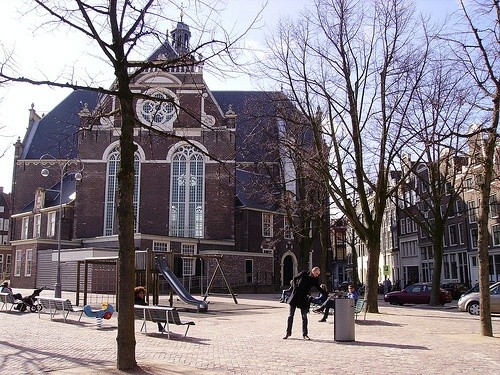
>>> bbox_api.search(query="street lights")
[40,154,86,298]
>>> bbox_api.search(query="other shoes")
[319,318,326,322]
[159,327,165,332]
[283,332,291,339]
[315,307,324,311]
[303,335,310,340]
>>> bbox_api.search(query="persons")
[0,281,22,309]
[14,289,39,310]
[387,279,391,293]
[134,286,166,332]
[308,284,329,305]
[396,280,400,290]
[279,280,293,303]
[283,267,335,340]
[315,285,359,322]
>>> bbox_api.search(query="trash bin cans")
[334,297,355,342]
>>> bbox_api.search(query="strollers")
[19,286,47,312]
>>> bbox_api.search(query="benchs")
[318,293,374,320]
[37,296,86,323]
[0,293,23,312]
[133,304,196,340]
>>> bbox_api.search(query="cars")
[457,281,500,315]
[442,283,460,300]
[461,283,499,297]
[383,283,452,306]
